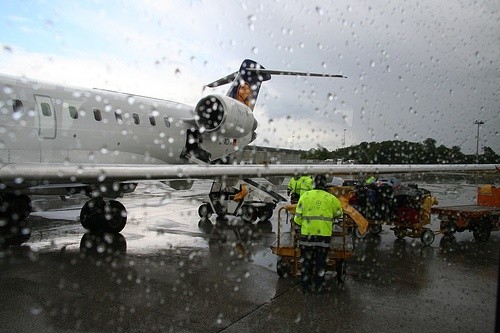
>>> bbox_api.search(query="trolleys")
[270,187,368,281]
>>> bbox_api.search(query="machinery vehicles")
[390,183,500,244]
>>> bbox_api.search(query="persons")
[295,175,343,293]
[287,172,300,204]
[365,175,380,184]
[297,175,313,198]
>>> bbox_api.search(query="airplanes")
[0,57,500,232]
